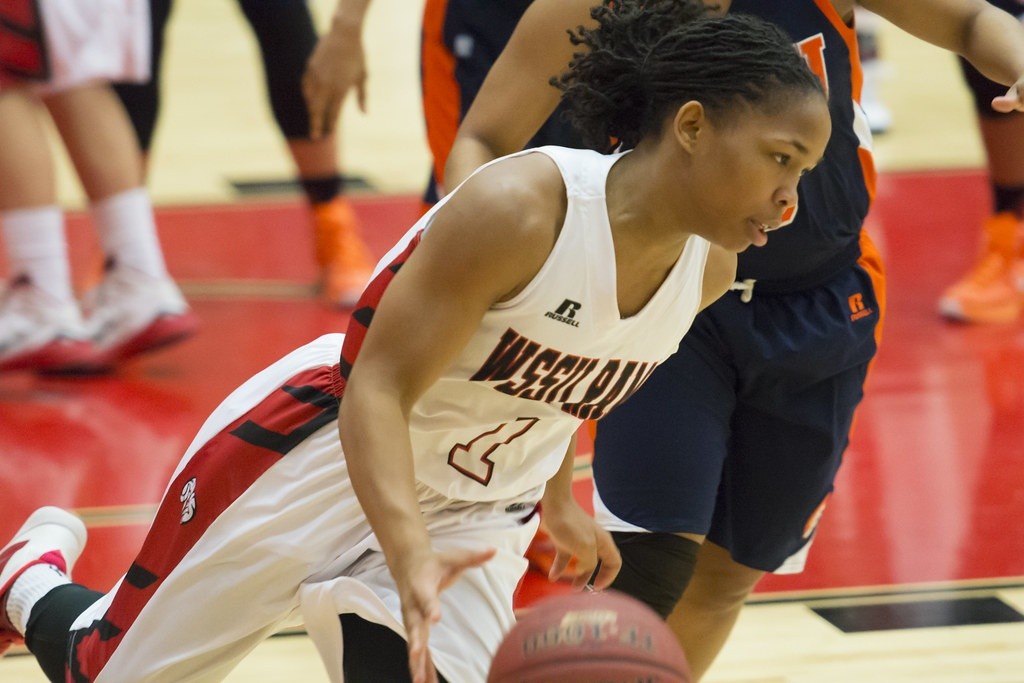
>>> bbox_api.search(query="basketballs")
[487,590,693,682]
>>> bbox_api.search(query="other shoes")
[0,271,90,372]
[938,213,1024,325]
[35,259,199,373]
[305,200,374,305]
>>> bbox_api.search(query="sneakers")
[0,506,89,657]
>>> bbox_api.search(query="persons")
[99,0,378,308]
[0,1,831,683]
[937,0,1024,323]
[443,0,1024,680]
[0,1,197,370]
[306,0,533,224]
